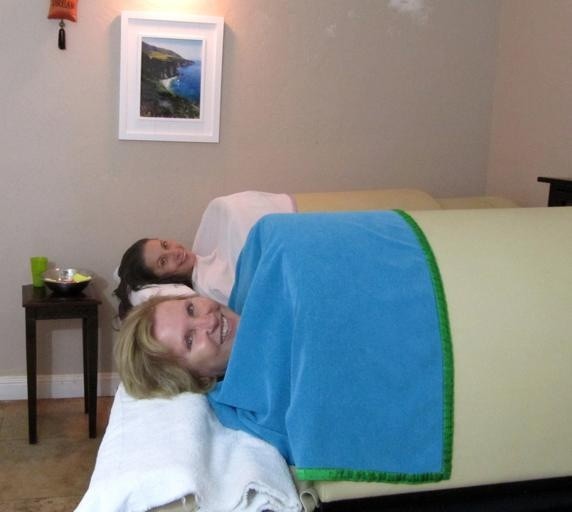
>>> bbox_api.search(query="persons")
[111,238,199,324]
[111,290,246,400]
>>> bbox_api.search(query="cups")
[31,256,48,287]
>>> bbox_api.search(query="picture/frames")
[116,11,225,146]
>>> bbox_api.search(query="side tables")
[20,280,102,444]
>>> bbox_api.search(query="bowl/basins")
[40,267,93,296]
